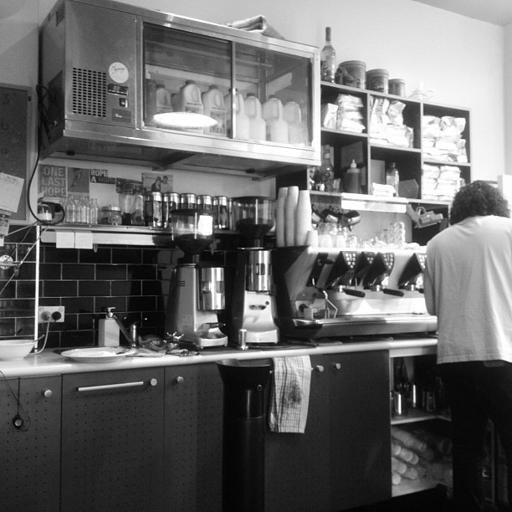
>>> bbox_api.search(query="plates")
[57,345,138,365]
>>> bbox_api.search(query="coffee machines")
[162,207,231,349]
[231,194,280,349]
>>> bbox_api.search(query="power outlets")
[39,306,65,323]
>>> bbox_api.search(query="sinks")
[53,346,153,358]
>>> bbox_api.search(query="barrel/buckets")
[222,87,247,138]
[155,82,173,112]
[243,92,266,142]
[281,96,308,147]
[261,94,289,143]
[202,83,226,137]
[178,79,203,115]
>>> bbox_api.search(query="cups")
[277,184,313,247]
[391,426,434,487]
[313,206,360,232]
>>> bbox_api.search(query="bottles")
[319,24,338,83]
[385,160,401,195]
[319,143,334,193]
[343,159,361,192]
[394,357,435,417]
[389,78,405,96]
[104,183,229,230]
[63,194,98,226]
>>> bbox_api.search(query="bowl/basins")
[0,338,36,363]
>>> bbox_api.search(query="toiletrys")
[98,306,120,347]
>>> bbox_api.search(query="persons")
[422,178,512,512]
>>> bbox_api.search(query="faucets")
[106,312,148,348]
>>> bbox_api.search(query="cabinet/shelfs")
[141,8,321,182]
[275,81,471,206]
